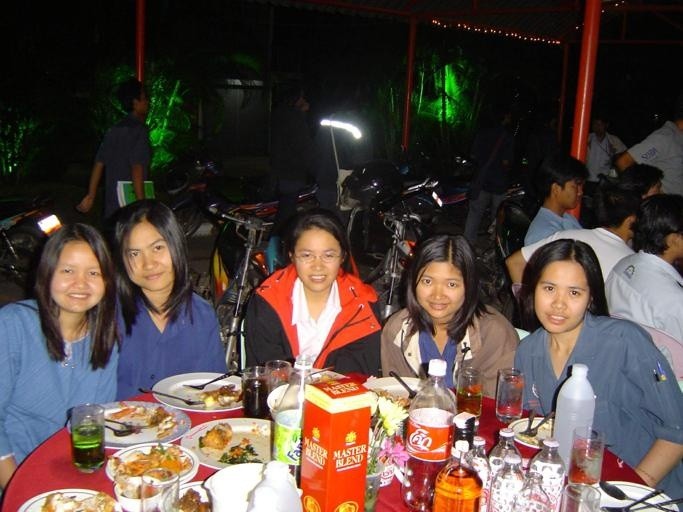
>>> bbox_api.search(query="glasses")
[290,252,344,264]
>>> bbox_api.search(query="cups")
[70,404,106,474]
[264,358,293,402]
[496,367,524,423]
[560,483,601,512]
[242,366,271,420]
[456,368,482,419]
[567,426,604,493]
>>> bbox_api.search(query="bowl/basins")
[114,476,163,512]
[267,382,304,427]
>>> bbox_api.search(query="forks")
[103,415,149,433]
[138,385,205,407]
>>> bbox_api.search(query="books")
[117,179,155,207]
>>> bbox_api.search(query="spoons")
[182,368,238,391]
[600,480,677,512]
[603,488,663,512]
[102,422,133,438]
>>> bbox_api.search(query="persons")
[504,182,642,305]
[524,151,591,247]
[0,222,120,494]
[585,115,628,182]
[457,105,515,243]
[380,231,521,399]
[615,162,664,200]
[76,77,156,218]
[242,207,382,377]
[615,91,683,194]
[512,238,683,512]
[263,81,318,200]
[114,198,230,407]
[604,192,682,348]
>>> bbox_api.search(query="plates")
[71,399,187,450]
[579,478,680,512]
[183,417,277,471]
[19,486,123,512]
[365,376,456,408]
[298,368,344,386]
[508,417,556,452]
[106,440,198,483]
[165,480,212,512]
[150,372,254,409]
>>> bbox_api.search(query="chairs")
[494,201,531,334]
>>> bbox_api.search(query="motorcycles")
[172,157,321,239]
[340,165,443,228]
[442,154,532,259]
[362,210,421,327]
[209,202,285,373]
[0,193,62,289]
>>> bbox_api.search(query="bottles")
[273,353,313,486]
[434,411,482,511]
[489,454,526,512]
[529,439,566,511]
[552,363,596,476]
[400,358,457,512]
[512,472,552,512]
[488,428,529,471]
[472,435,487,467]
